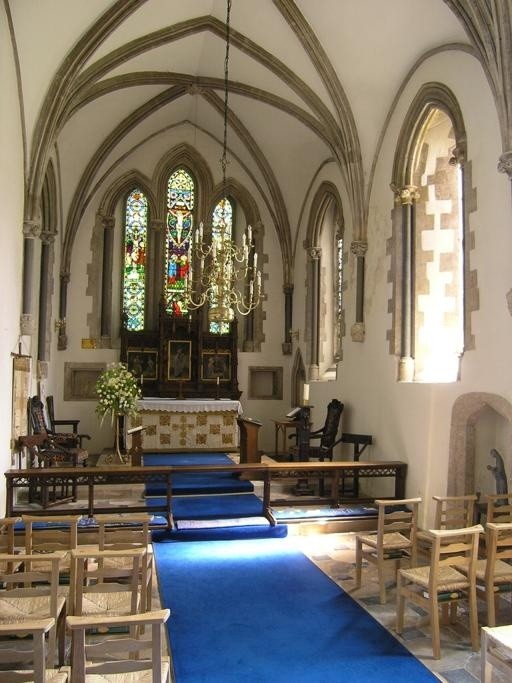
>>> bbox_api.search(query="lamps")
[184,0,265,336]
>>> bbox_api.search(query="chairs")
[284,398,372,499]
[355,494,512,683]
[0,513,171,683]
[19,396,90,508]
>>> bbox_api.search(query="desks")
[262,462,413,526]
[123,397,243,454]
[273,419,313,456]
[5,465,175,530]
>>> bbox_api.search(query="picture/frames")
[127,340,232,382]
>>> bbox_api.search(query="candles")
[141,374,143,384]
[217,377,220,384]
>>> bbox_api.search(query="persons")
[486,447,508,507]
[179,255,189,285]
[174,348,186,377]
[169,209,191,245]
[208,358,216,376]
[169,253,181,284]
[133,356,144,373]
[147,355,154,372]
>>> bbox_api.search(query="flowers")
[95,361,144,430]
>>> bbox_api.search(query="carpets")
[176,516,270,529]
